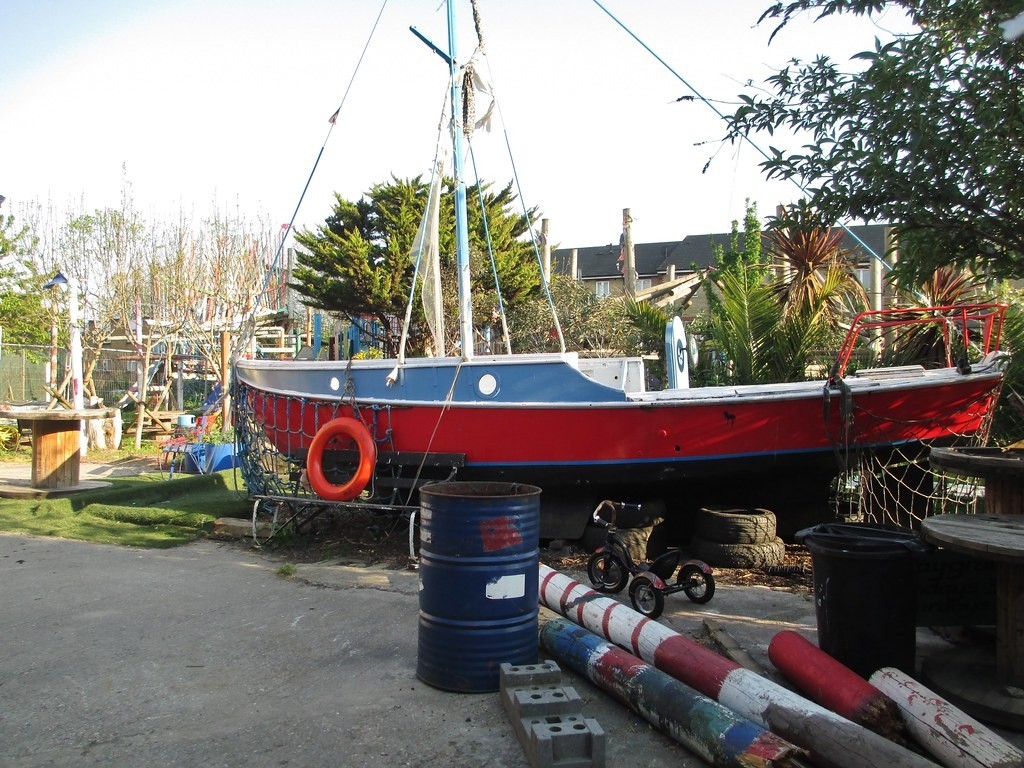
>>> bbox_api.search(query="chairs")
[162,415,205,479]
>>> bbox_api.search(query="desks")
[0,410,115,488]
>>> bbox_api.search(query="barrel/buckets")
[796,522,922,680]
[416,481,543,694]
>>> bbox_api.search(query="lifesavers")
[305,416,377,502]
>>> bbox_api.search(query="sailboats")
[229,0,1010,541]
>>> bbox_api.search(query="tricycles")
[588,499,714,621]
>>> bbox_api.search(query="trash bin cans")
[178,415,196,428]
[862,443,935,523]
[795,522,946,680]
